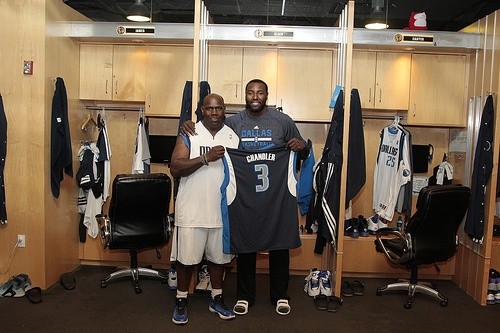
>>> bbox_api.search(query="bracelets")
[201,154,209,166]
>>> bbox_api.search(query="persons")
[170,93,244,324]
[180,79,311,315]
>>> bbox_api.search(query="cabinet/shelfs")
[71,42,193,280]
[342,49,472,278]
[207,44,328,276]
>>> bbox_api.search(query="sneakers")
[167,270,179,290]
[367,215,392,236]
[171,299,190,325]
[0,274,33,297]
[209,295,236,321]
[304,267,333,297]
[345,214,369,240]
[196,266,226,292]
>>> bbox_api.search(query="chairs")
[96,173,172,295]
[374,184,471,309]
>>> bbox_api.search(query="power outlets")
[18,235,25,247]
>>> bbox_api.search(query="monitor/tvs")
[411,144,429,174]
[147,135,177,164]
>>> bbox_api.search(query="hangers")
[389,116,410,134]
[137,108,147,125]
[82,106,97,130]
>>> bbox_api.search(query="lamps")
[365,0,389,30]
[127,0,150,22]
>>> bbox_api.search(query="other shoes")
[486,292,500,305]
[488,268,500,291]
[26,287,45,304]
[275,297,293,316]
[313,294,344,313]
[341,280,365,297]
[61,271,77,291]
[233,299,250,316]
